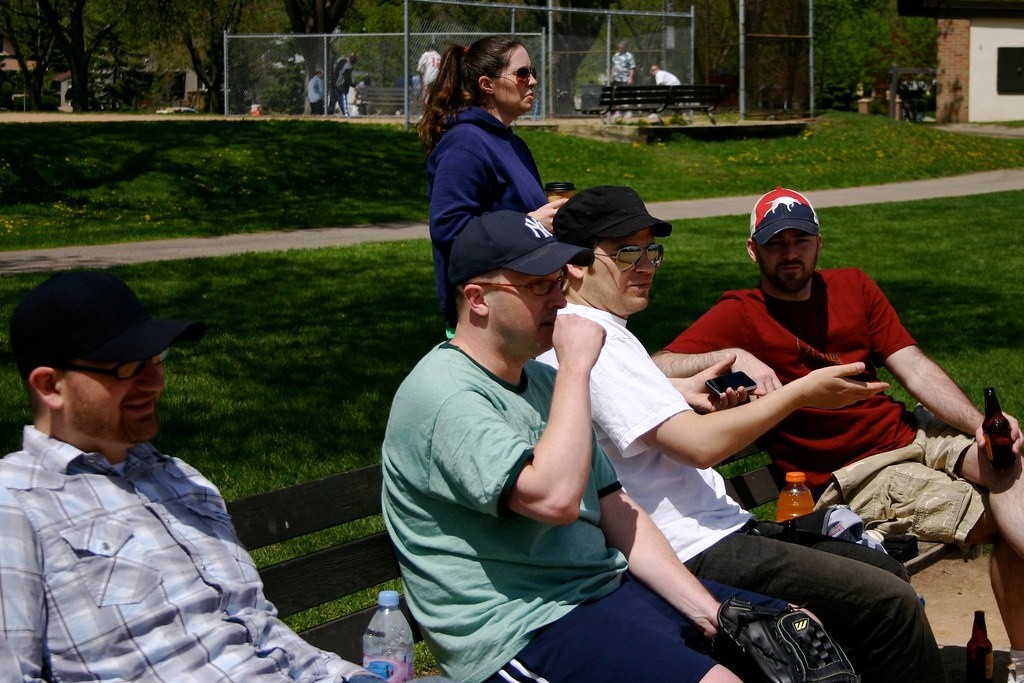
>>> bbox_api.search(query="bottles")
[982,387,1016,470]
[968,611,993,683]
[777,472,814,524]
[917,593,925,611]
[362,590,414,683]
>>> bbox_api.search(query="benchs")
[573,81,730,126]
[360,86,414,116]
[223,363,963,683]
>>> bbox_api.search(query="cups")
[543,183,577,211]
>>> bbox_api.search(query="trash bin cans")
[188,91,205,112]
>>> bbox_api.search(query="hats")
[447,211,595,288]
[8,270,208,380]
[749,187,820,247]
[550,186,673,247]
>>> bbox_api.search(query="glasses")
[464,275,573,297]
[593,243,663,273]
[47,345,170,381]
[489,63,537,85]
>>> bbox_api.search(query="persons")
[419,36,574,342]
[536,184,945,682]
[307,69,324,115]
[381,212,825,683]
[353,75,375,116]
[393,42,442,115]
[540,54,574,115]
[609,42,636,118]
[650,65,681,117]
[653,186,1024,683]
[0,268,389,683]
[327,52,358,117]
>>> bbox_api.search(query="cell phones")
[705,371,757,397]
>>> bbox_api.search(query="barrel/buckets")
[250,104,263,116]
